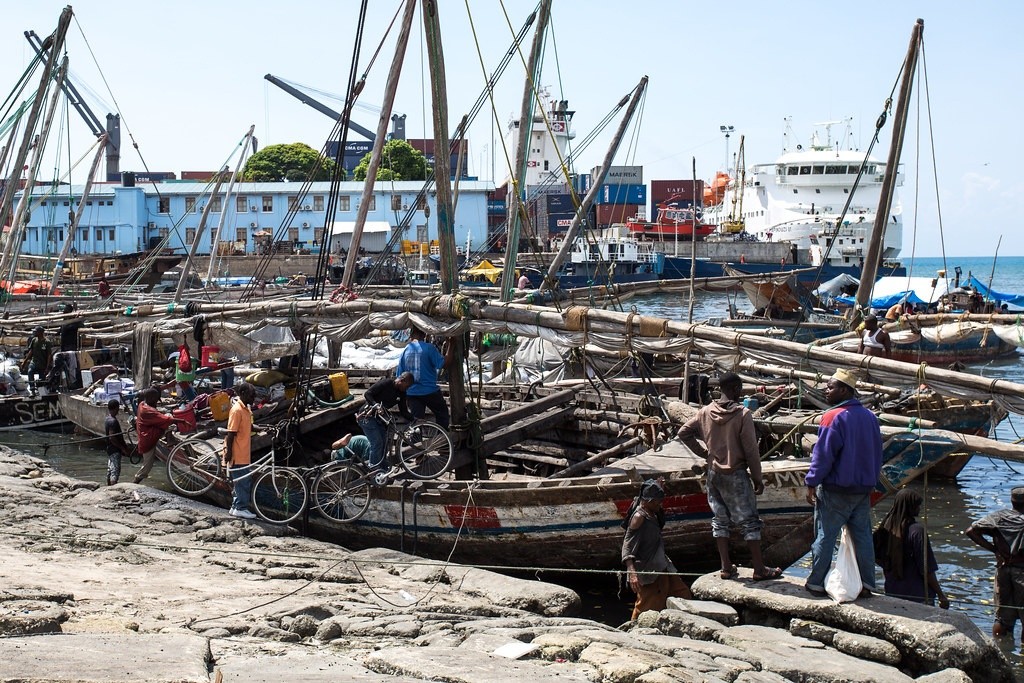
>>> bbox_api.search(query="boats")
[842,321,1019,362]
[58,247,182,293]
[0,377,77,434]
[805,308,847,340]
[895,390,1009,478]
[56,373,170,446]
[655,252,908,285]
[940,288,981,313]
[0,0,964,581]
[523,226,658,289]
[625,208,719,239]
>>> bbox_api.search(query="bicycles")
[165,418,308,525]
[310,400,454,525]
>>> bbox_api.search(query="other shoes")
[29,393,35,397]
[860,590,871,598]
[805,583,826,597]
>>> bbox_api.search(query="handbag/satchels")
[179,334,193,373]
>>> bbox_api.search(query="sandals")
[753,567,783,581]
[720,564,737,579]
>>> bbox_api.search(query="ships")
[702,116,905,266]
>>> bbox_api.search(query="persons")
[132,389,192,484]
[857,314,892,359]
[873,489,949,610]
[224,383,269,518]
[330,324,457,480]
[804,367,883,598]
[20,304,78,399]
[885,298,953,323]
[965,486,1024,645]
[546,238,557,252]
[337,241,345,253]
[621,478,693,621]
[291,271,303,285]
[1001,304,1010,314]
[517,272,534,290]
[676,372,782,581]
[99,276,110,297]
[105,399,131,486]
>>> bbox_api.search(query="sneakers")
[228,508,235,515]
[233,508,257,519]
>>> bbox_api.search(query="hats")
[31,326,44,332]
[1010,487,1023,503]
[642,484,665,500]
[832,367,859,388]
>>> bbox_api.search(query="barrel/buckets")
[744,398,758,411]
[172,404,197,435]
[201,346,220,367]
[208,391,230,421]
[312,372,349,403]
[175,356,196,381]
